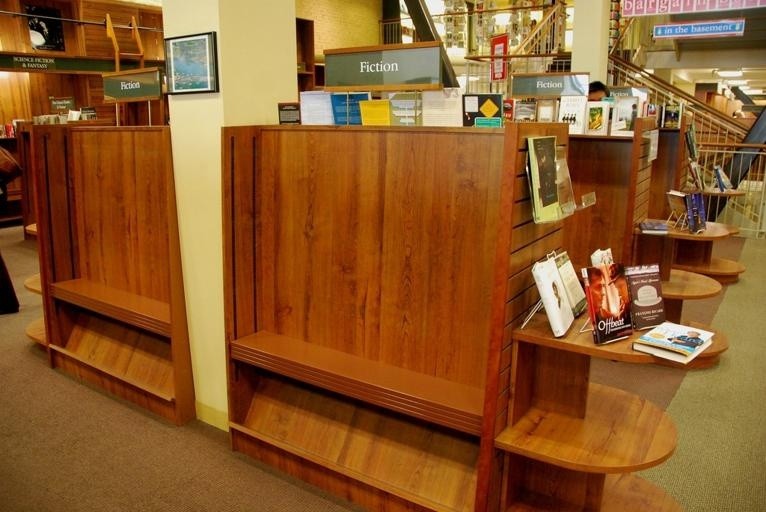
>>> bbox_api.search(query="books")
[504,95,587,136]
[647,104,682,129]
[554,245,590,316]
[279,91,373,125]
[529,257,575,340]
[360,88,504,126]
[525,132,561,222]
[666,189,696,233]
[685,130,733,191]
[624,262,666,328]
[587,87,650,136]
[9,104,98,141]
[639,221,670,235]
[632,321,716,367]
[578,260,634,347]
[690,191,707,231]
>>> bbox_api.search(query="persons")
[588,82,610,100]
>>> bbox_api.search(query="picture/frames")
[163,30,220,95]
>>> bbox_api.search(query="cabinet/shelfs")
[296,18,315,103]
[16,89,747,512]
